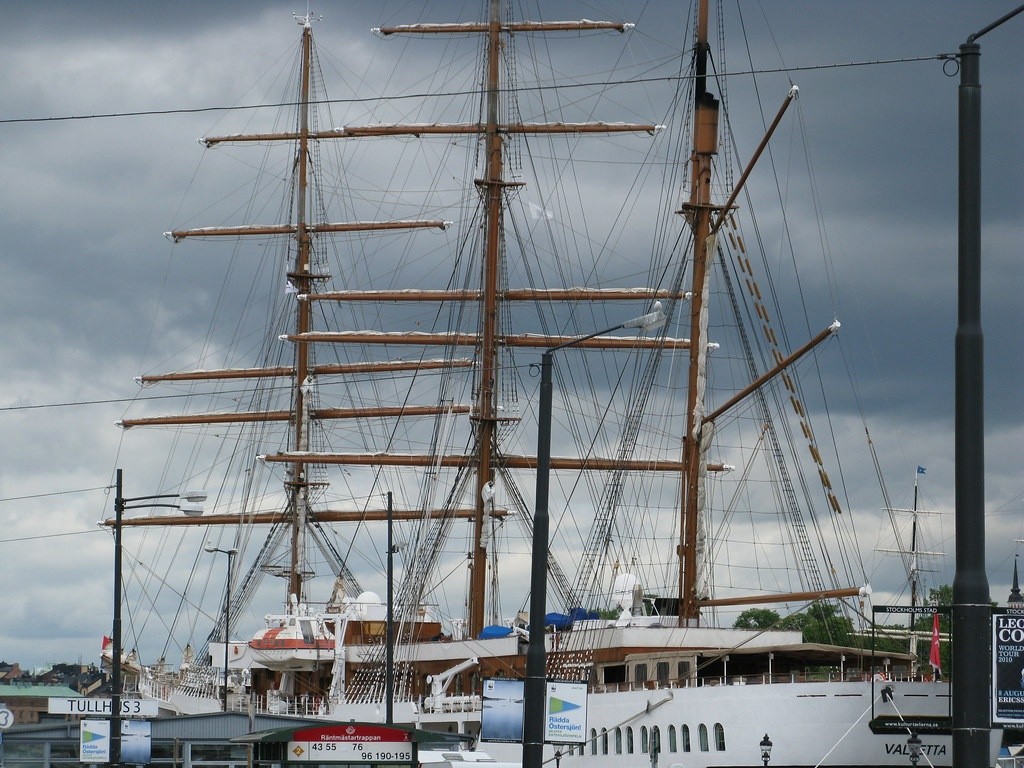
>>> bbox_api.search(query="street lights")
[386,542,408,726]
[203,545,238,713]
[109,467,207,766]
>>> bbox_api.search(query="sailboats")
[2,0,1024,766]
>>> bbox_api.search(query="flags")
[529,202,553,220]
[929,614,942,674]
